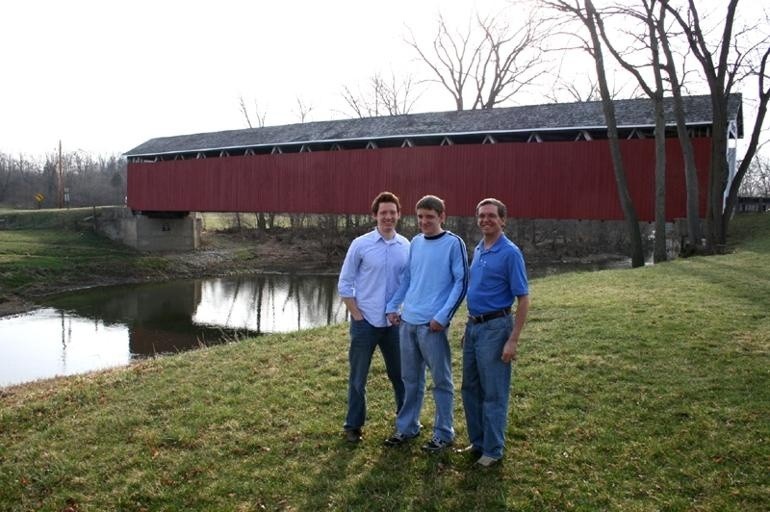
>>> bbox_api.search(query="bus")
[724,195,767,213]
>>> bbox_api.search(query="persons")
[454,199,529,472]
[384,196,468,452]
[338,191,411,443]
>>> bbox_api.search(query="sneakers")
[422,436,448,449]
[384,432,407,444]
[472,455,500,470]
[456,444,480,454]
[347,431,359,444]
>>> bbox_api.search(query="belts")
[468,308,512,324]
